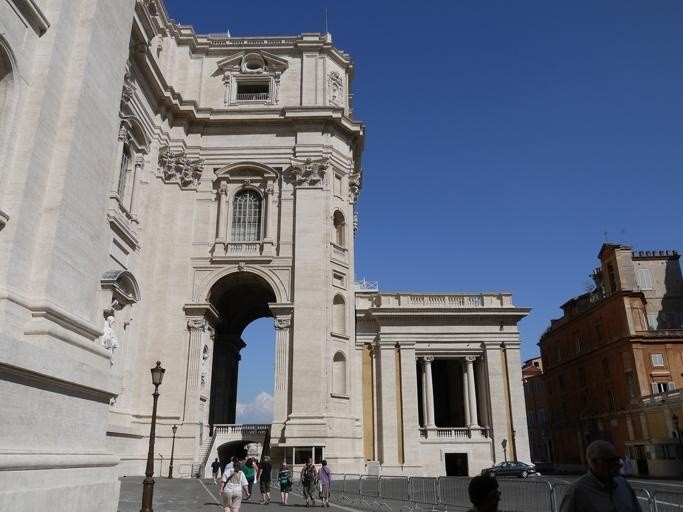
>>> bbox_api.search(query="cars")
[484,460,536,478]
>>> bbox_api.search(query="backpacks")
[303,464,314,487]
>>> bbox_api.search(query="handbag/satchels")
[316,480,322,493]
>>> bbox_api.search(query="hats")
[264,456,270,464]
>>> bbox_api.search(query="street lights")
[140,360,166,511]
[167,425,178,479]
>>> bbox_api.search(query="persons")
[211,456,259,512]
[104,316,120,349]
[301,457,318,508]
[559,441,643,512]
[466,475,501,512]
[277,464,291,504]
[317,460,331,507]
[258,455,272,505]
